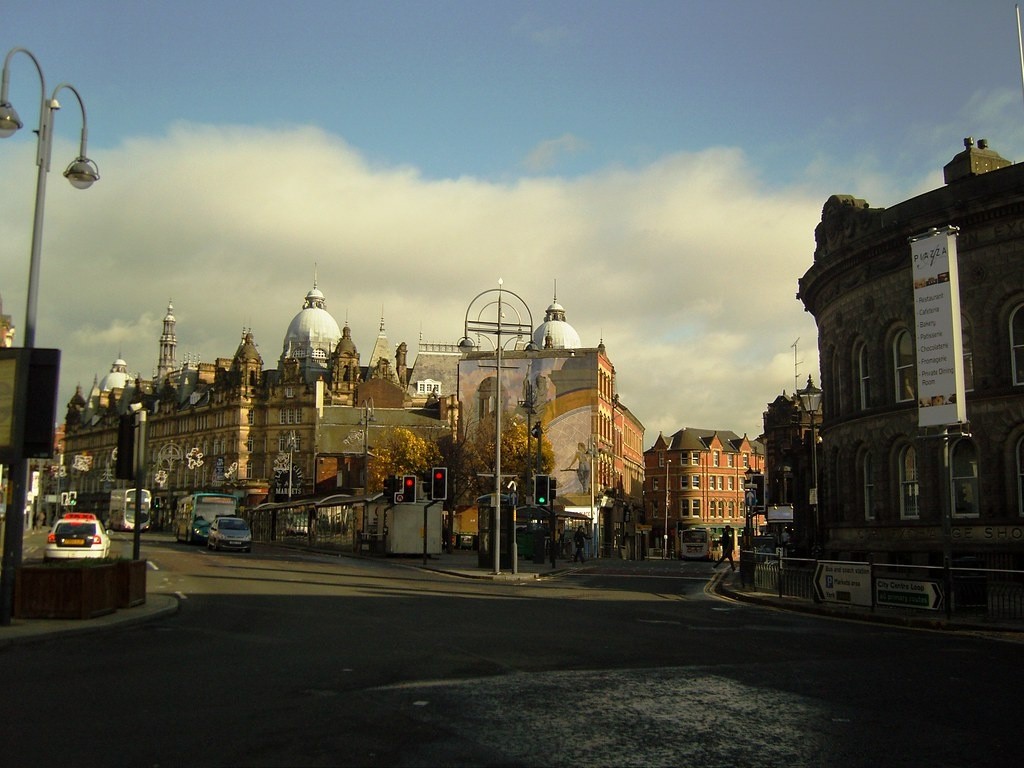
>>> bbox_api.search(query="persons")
[32,511,45,534]
[574,525,593,564]
[711,525,741,573]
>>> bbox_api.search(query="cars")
[43,518,114,559]
[207,517,252,552]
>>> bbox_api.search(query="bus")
[108,489,152,532]
[678,529,709,561]
[173,493,237,545]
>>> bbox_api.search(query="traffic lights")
[549,478,557,501]
[383,475,396,506]
[61,492,68,506]
[403,476,416,504]
[431,468,447,500]
[422,470,431,500]
[534,475,549,506]
[68,491,78,506]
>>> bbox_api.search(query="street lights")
[357,396,377,494]
[0,47,101,624]
[797,375,825,603]
[457,277,539,576]
[744,465,758,546]
[665,460,671,559]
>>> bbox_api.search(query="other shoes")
[712,567,717,572]
[734,570,740,572]
